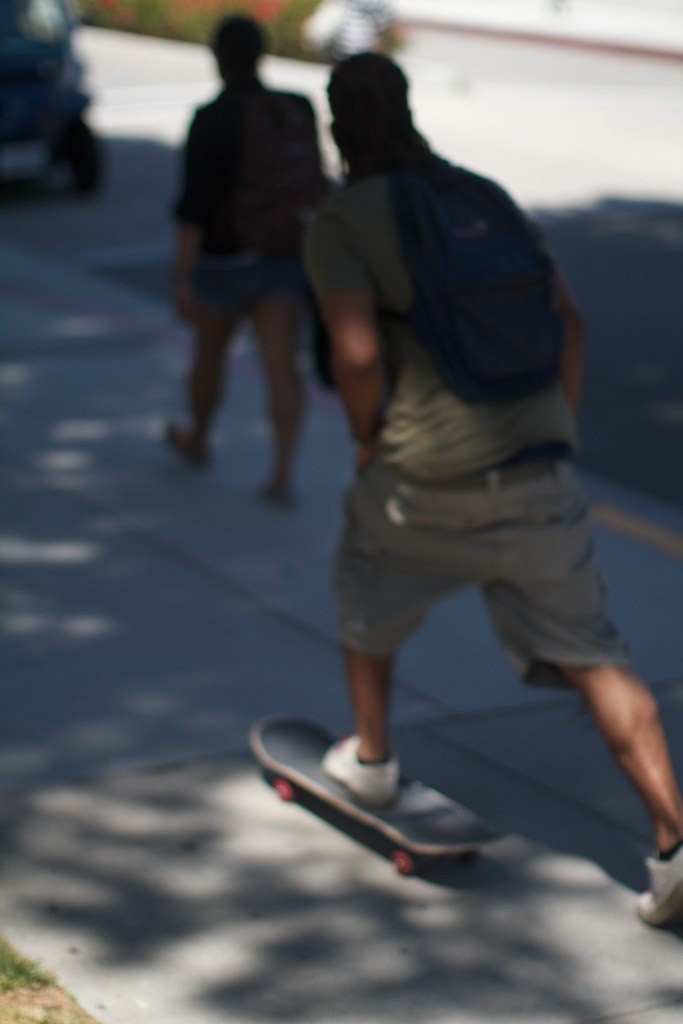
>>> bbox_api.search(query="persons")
[162,16,324,503]
[304,1,396,61]
[298,50,682,929]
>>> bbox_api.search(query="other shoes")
[259,483,296,507]
[632,840,683,924]
[321,732,400,804]
[169,425,212,466]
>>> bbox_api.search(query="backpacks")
[337,154,568,402]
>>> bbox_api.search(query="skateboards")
[248,711,500,872]
